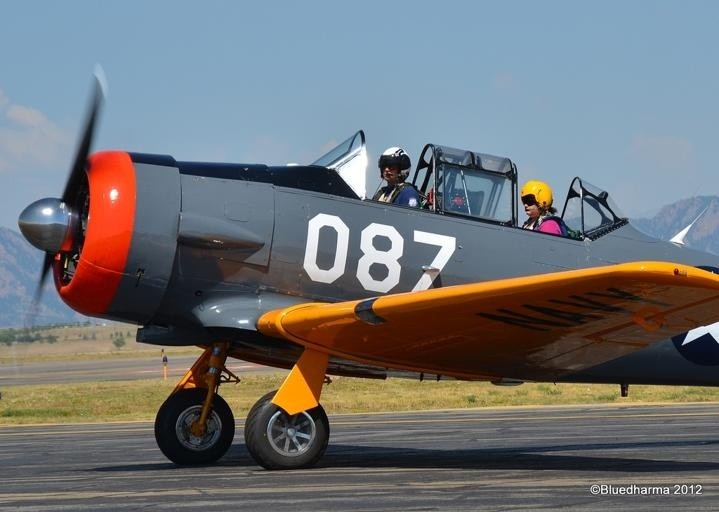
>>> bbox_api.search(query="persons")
[519,179,573,237]
[369,143,427,211]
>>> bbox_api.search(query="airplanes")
[17,63,719,470]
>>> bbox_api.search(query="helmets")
[378,147,411,183]
[520,181,553,211]
